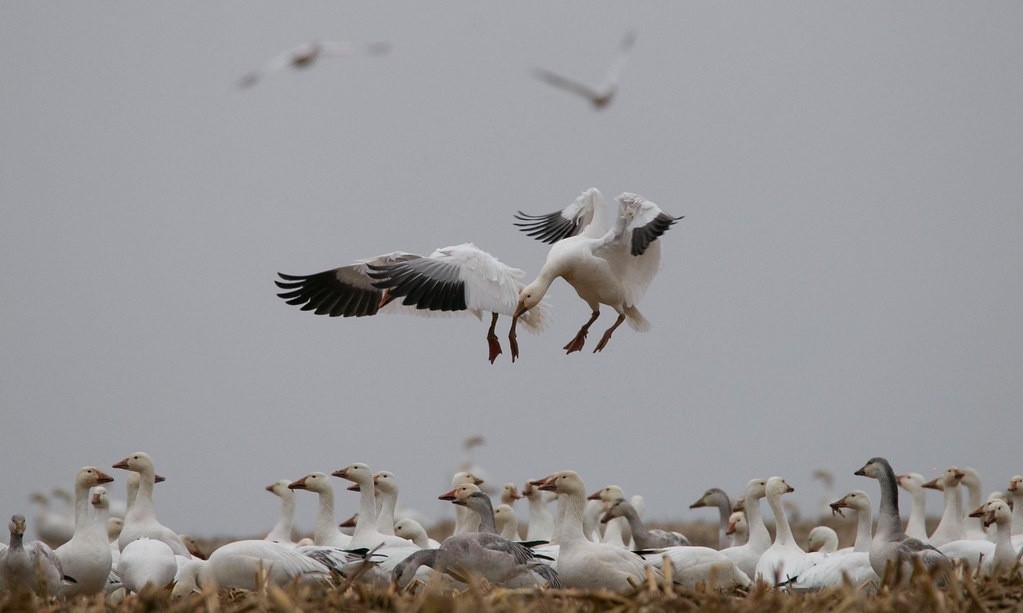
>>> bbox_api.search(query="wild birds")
[274,242,554,365]
[44,452,1023,598]
[512,188,685,356]
[0,512,78,609]
